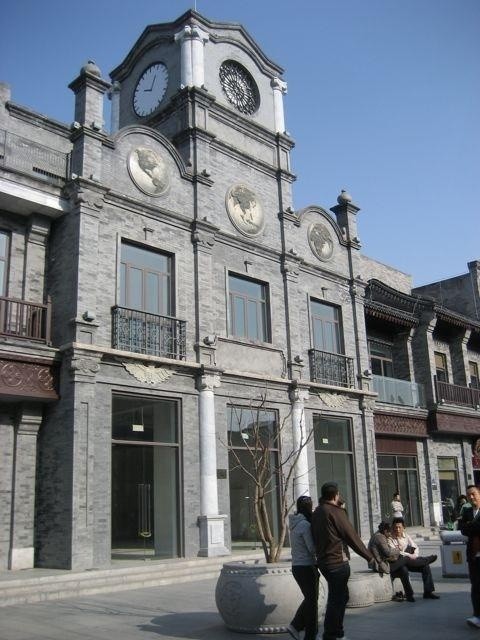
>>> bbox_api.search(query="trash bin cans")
[440,530,468,578]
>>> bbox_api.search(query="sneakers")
[406,592,416,603]
[424,554,437,563]
[394,591,404,601]
[422,592,439,599]
[287,623,300,640]
[466,614,480,629]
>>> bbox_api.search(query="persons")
[460,485,480,628]
[390,493,406,530]
[367,520,426,602]
[310,481,375,640]
[387,517,440,599]
[337,499,350,561]
[286,495,321,640]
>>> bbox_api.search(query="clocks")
[131,60,169,118]
